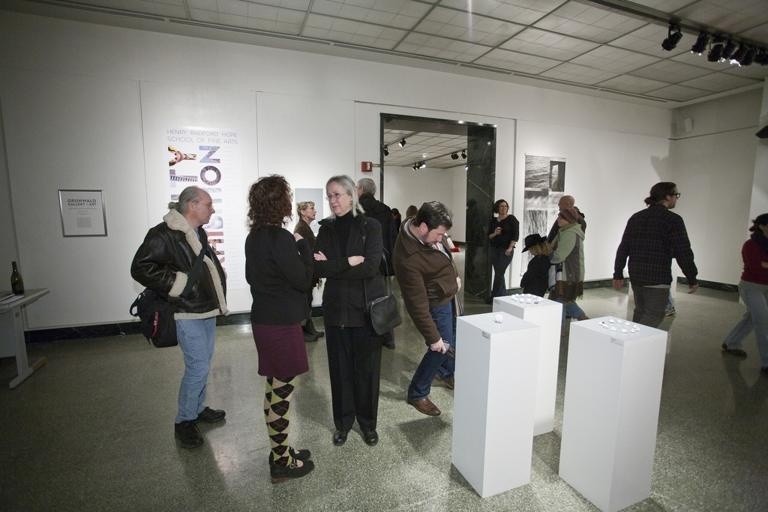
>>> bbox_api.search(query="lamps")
[451,152,466,160]
[662,31,768,67]
[399,140,406,147]
[412,161,426,171]
[384,146,389,156]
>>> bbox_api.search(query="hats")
[522,233,547,252]
[558,206,579,224]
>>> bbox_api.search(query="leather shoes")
[408,397,442,417]
[434,373,454,389]
[333,430,349,446]
[362,429,379,446]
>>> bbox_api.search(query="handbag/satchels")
[367,294,402,336]
[130,290,177,347]
[555,280,583,298]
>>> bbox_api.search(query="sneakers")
[722,340,746,356]
[198,407,225,423]
[174,420,203,449]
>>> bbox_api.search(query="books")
[0,293,25,305]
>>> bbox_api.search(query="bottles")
[10,261,24,295]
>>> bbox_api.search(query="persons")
[613,181,699,330]
[721,212,768,379]
[391,201,461,416]
[245,176,314,478]
[354,178,397,349]
[294,202,325,343]
[130,186,229,449]
[392,205,417,230]
[486,199,519,303]
[521,194,587,336]
[313,176,383,444]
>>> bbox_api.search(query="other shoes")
[269,449,311,464]
[303,319,324,343]
[270,460,314,478]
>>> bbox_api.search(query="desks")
[0,287,50,389]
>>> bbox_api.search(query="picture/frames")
[58,189,107,237]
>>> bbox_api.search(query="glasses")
[670,192,680,199]
